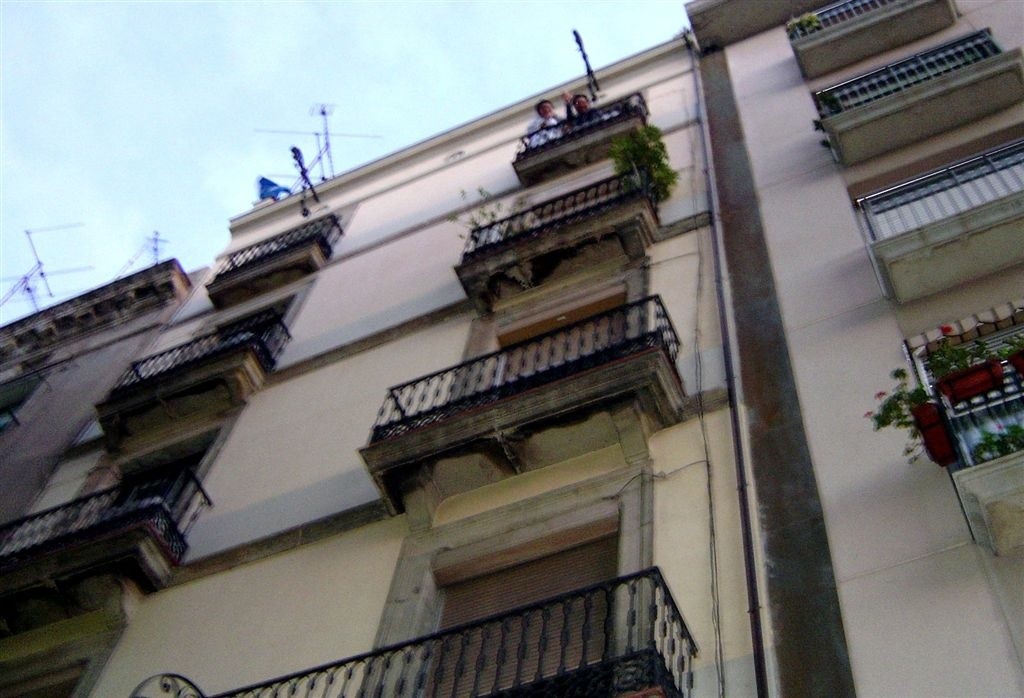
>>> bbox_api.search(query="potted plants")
[997,332,1024,380]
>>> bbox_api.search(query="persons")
[526,91,605,151]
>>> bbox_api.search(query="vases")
[912,402,958,468]
[934,357,1003,404]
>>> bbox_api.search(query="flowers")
[865,368,925,465]
[925,325,993,380]
